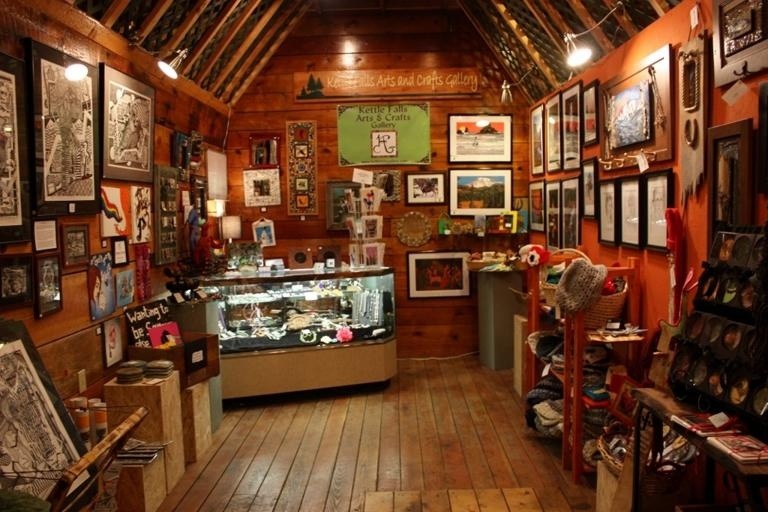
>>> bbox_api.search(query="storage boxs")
[130,334,220,387]
[592,463,674,512]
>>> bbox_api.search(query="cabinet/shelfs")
[530,256,639,480]
[632,386,767,512]
[186,263,397,412]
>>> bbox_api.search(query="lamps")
[58,37,88,82]
[127,33,189,79]
[501,63,538,103]
[564,2,623,66]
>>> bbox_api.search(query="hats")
[554,257,608,313]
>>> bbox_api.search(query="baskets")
[539,244,593,307]
[466,251,529,270]
[596,431,684,496]
[540,272,630,330]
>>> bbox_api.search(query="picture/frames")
[545,179,561,253]
[544,90,562,174]
[645,170,674,255]
[598,178,618,248]
[0,252,33,310]
[404,170,447,207]
[286,120,318,215]
[448,113,513,164]
[619,175,644,251]
[581,80,600,148]
[324,179,361,234]
[530,102,545,175]
[705,116,755,227]
[317,243,342,268]
[372,171,401,203]
[0,52,33,246]
[287,246,313,269]
[580,155,598,219]
[58,222,91,275]
[561,80,583,171]
[527,179,544,231]
[0,320,98,512]
[111,236,130,267]
[560,176,582,249]
[597,44,673,171]
[22,37,103,218]
[406,249,472,300]
[98,62,154,184]
[499,210,517,234]
[32,215,57,252]
[35,250,65,319]
[711,0,768,89]
[101,317,124,368]
[448,167,513,218]
[247,132,281,169]
